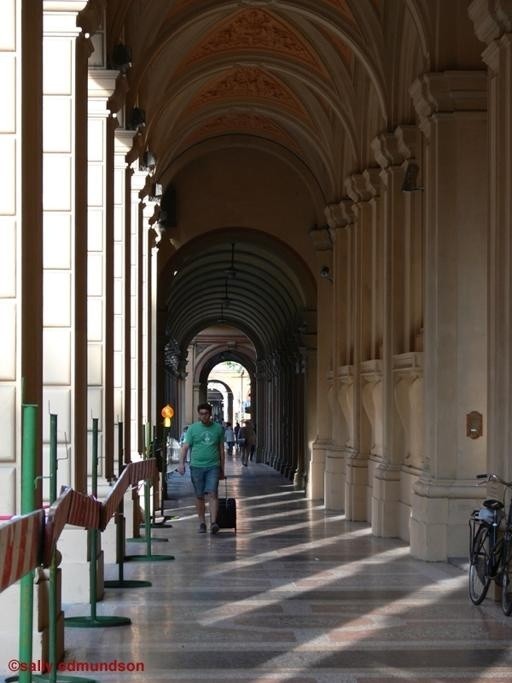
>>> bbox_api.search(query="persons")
[218,418,256,467]
[178,404,225,533]
[180,427,189,464]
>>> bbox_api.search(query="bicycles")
[457,471,512,619]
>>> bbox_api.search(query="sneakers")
[209,522,219,534]
[200,523,207,533]
[241,462,247,467]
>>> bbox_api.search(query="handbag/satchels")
[238,438,247,447]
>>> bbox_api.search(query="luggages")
[215,497,236,533]
